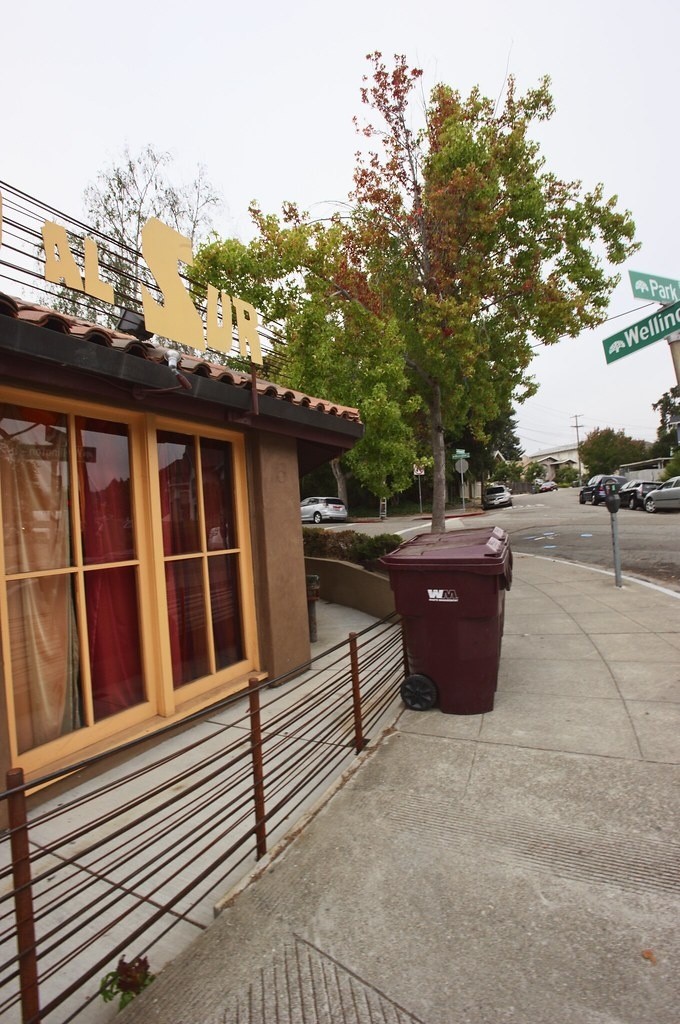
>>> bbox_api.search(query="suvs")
[300,497,348,524]
[579,474,629,505]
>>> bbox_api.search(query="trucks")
[532,479,544,487]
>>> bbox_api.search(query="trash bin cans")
[378,527,513,714]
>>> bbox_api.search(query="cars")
[617,479,666,510]
[539,482,557,493]
[644,476,680,513]
[483,485,512,511]
